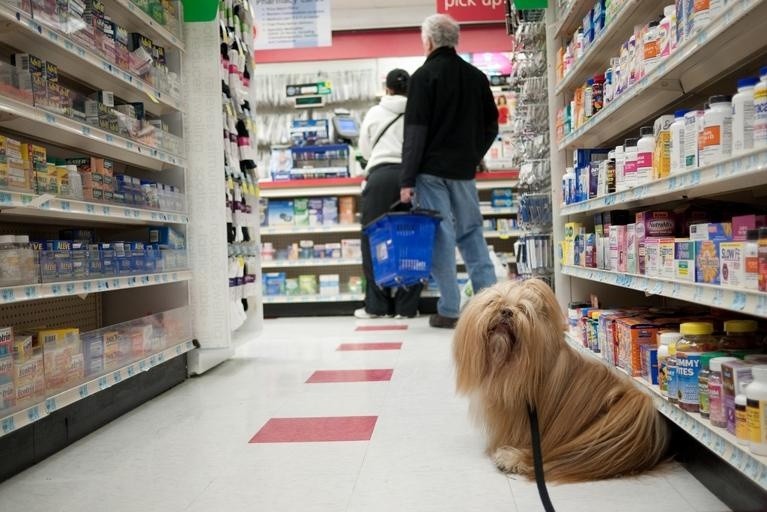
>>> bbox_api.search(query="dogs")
[451,278,670,487]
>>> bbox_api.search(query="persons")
[352,67,425,319]
[398,14,500,329]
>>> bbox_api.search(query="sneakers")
[428,315,457,328]
[395,312,421,320]
[353,308,393,320]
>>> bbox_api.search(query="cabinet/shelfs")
[0,0,191,484]
[506,1,555,301]
[257,166,531,307]
[550,0,767,511]
[181,0,265,381]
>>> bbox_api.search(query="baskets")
[362,200,443,290]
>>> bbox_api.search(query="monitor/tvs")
[332,117,360,138]
